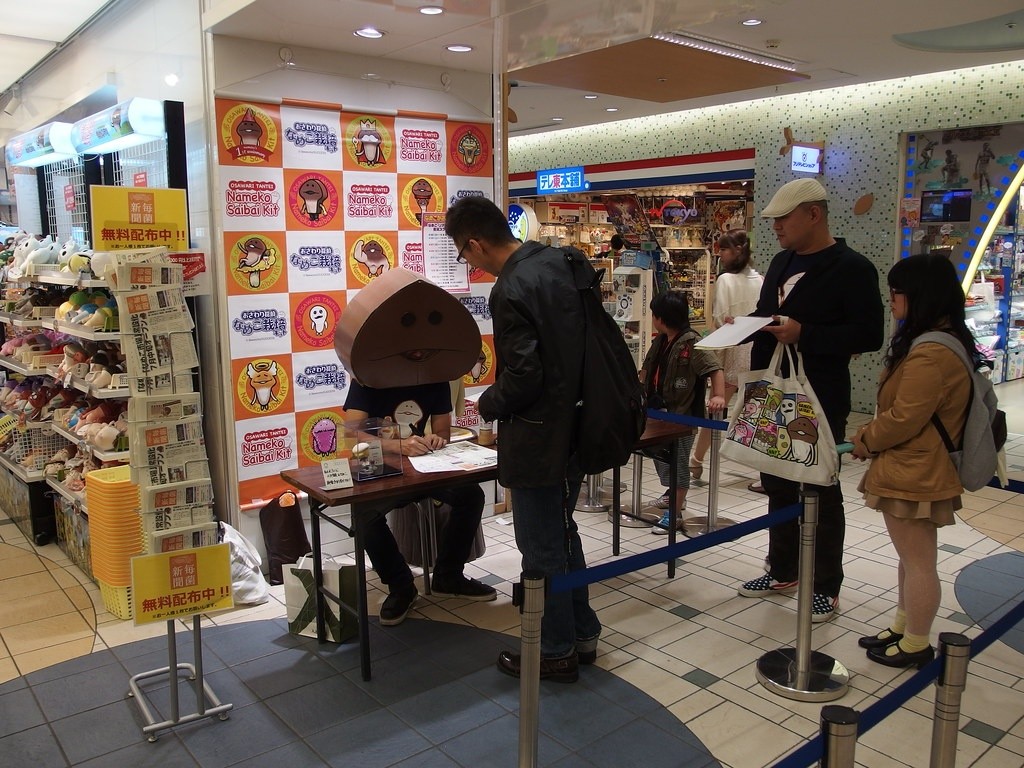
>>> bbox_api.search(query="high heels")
[748,482,766,493]
[858,627,904,648]
[687,466,703,479]
[866,640,935,671]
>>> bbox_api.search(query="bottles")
[478,413,495,445]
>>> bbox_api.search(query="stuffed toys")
[1,230,128,491]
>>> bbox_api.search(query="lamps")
[4,82,21,116]
[636,184,707,198]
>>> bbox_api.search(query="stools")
[390,497,487,567]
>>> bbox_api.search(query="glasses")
[456,239,478,264]
[890,287,906,302]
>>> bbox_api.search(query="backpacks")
[559,246,648,477]
[906,331,1010,493]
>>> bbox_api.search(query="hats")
[761,177,828,220]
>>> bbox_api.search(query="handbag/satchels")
[219,521,271,604]
[720,317,841,488]
[258,492,312,586]
[281,552,357,643]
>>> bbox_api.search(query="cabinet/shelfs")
[962,229,1024,386]
[531,197,754,385]
[0,248,235,745]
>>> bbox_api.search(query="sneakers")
[738,572,798,598]
[811,593,839,622]
[652,511,684,534]
[648,489,687,509]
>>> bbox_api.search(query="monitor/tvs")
[919,189,972,223]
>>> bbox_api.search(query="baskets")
[86,464,149,621]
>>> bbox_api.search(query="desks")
[281,417,692,682]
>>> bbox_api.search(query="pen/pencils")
[409,423,433,452]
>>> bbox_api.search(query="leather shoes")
[575,649,597,665]
[497,651,579,685]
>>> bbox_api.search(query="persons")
[334,265,497,625]
[722,177,885,623]
[684,229,767,494]
[919,141,995,195]
[447,196,603,683]
[640,289,724,533]
[848,254,987,669]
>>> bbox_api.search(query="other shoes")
[430,566,496,602]
[380,582,419,625]
